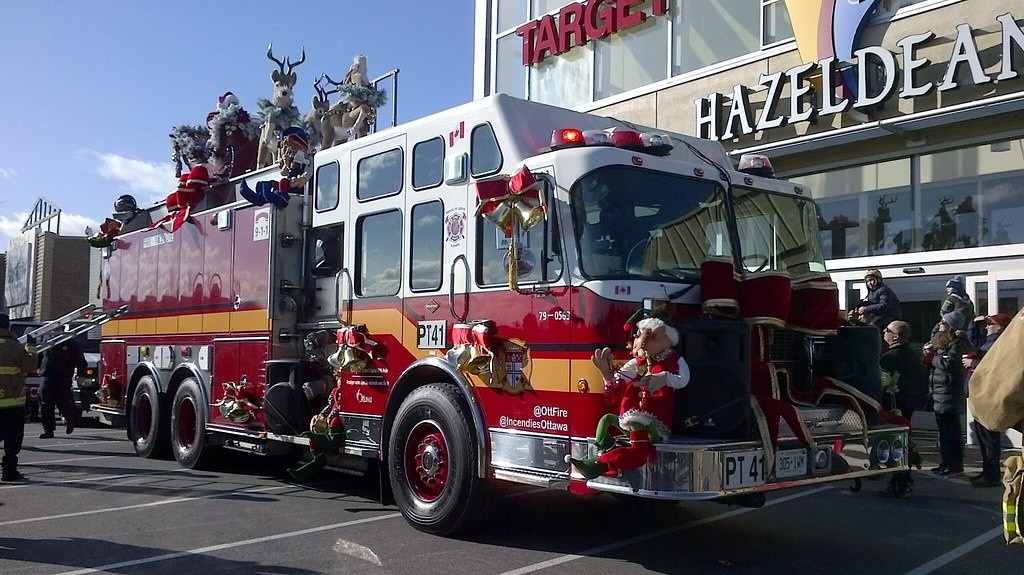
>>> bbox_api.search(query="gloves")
[27,333,37,343]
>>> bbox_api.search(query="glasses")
[941,322,950,327]
[987,322,996,326]
[858,312,868,316]
[886,328,900,336]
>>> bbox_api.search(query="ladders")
[17,302,131,354]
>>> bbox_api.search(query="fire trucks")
[92,91,913,533]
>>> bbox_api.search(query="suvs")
[8,321,102,428]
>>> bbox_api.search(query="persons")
[240,127,314,209]
[0,314,38,485]
[848,269,903,353]
[922,275,974,357]
[287,368,347,483]
[564,305,690,480]
[968,305,1024,547]
[870,321,922,498]
[923,311,974,477]
[962,314,1012,488]
[37,326,88,439]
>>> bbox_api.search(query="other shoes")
[66,423,75,434]
[968,473,1003,488]
[38,431,54,439]
[1,473,25,481]
[880,486,912,498]
[931,467,964,477]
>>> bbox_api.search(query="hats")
[0,312,10,328]
[865,269,883,290]
[945,274,964,290]
[987,313,1012,328]
[942,311,965,331]
